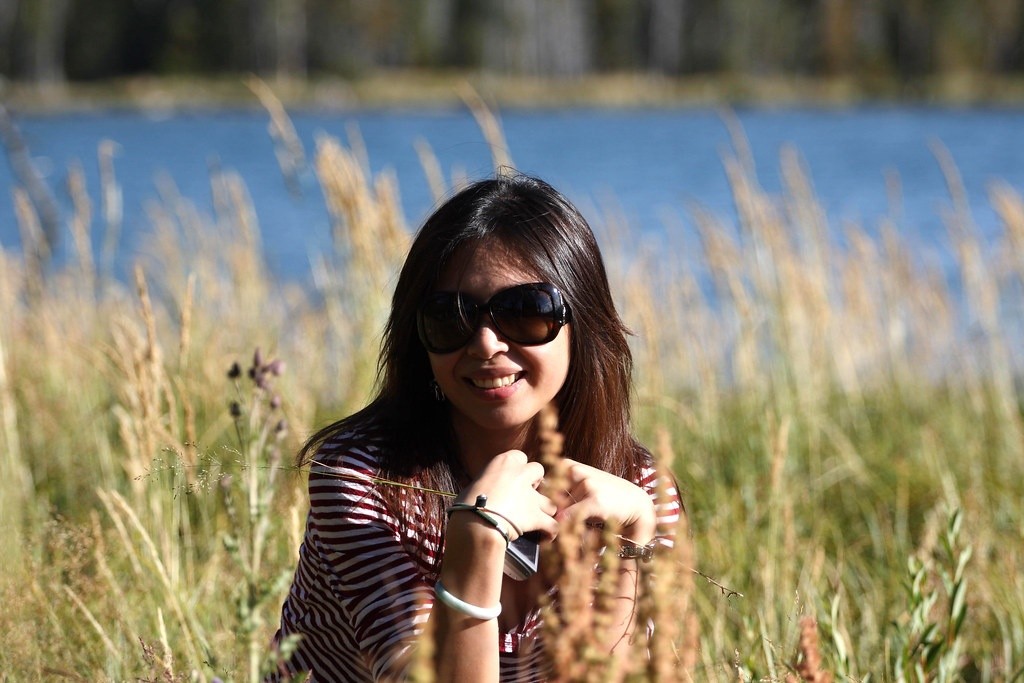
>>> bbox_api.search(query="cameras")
[504,532,539,582]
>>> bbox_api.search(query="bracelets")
[435,583,502,620]
[446,495,523,544]
[618,541,656,563]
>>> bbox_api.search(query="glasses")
[417,279,574,356]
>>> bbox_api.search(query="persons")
[265,176,680,683]
